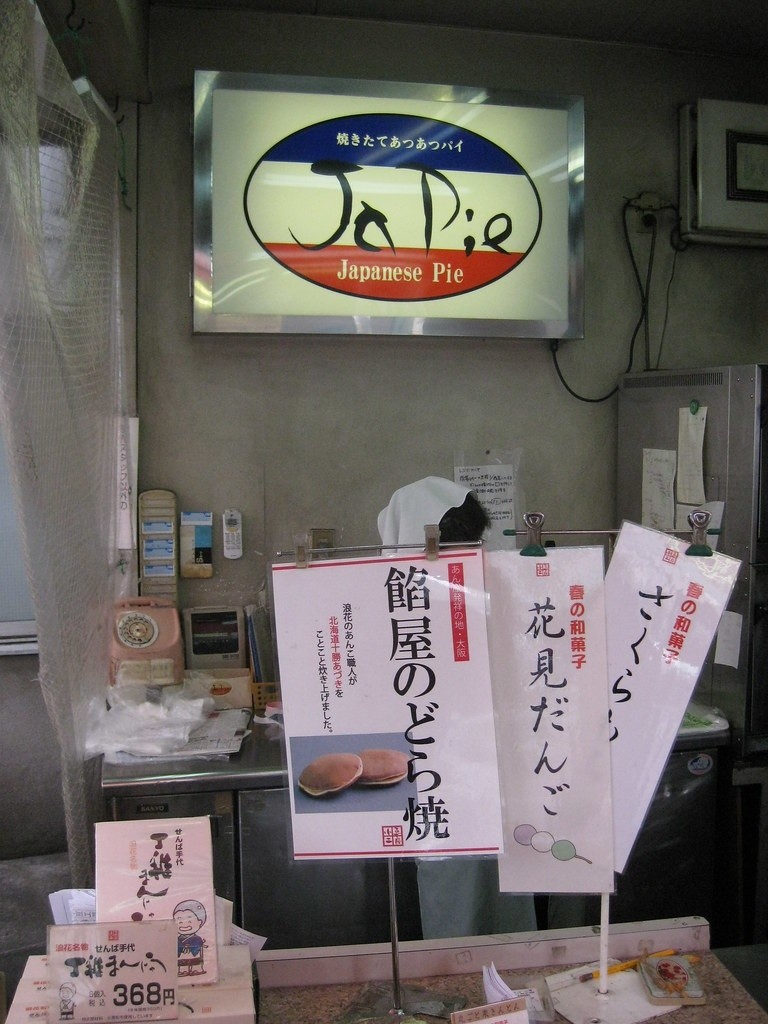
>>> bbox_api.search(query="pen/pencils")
[579,947,682,983]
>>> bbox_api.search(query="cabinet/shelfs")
[97,699,742,951]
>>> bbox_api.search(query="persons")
[373,474,539,940]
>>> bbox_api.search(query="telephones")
[106,596,185,688]
[222,508,243,560]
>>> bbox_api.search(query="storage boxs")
[181,667,254,710]
